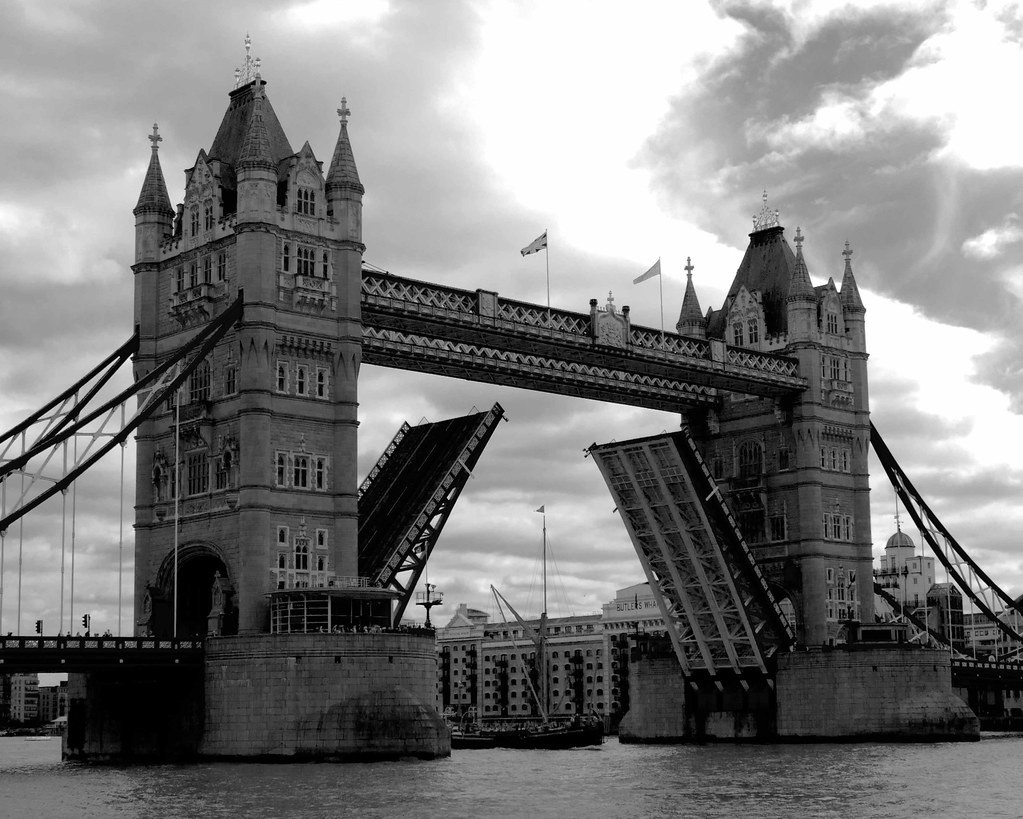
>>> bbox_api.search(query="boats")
[442,515,605,750]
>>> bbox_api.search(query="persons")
[926,639,932,647]
[464,712,597,734]
[141,631,155,637]
[822,641,828,648]
[318,622,435,633]
[1013,657,1019,663]
[934,643,946,649]
[7,632,12,636]
[58,629,112,637]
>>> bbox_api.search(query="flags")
[520,232,547,257]
[534,506,545,513]
[633,259,660,284]
